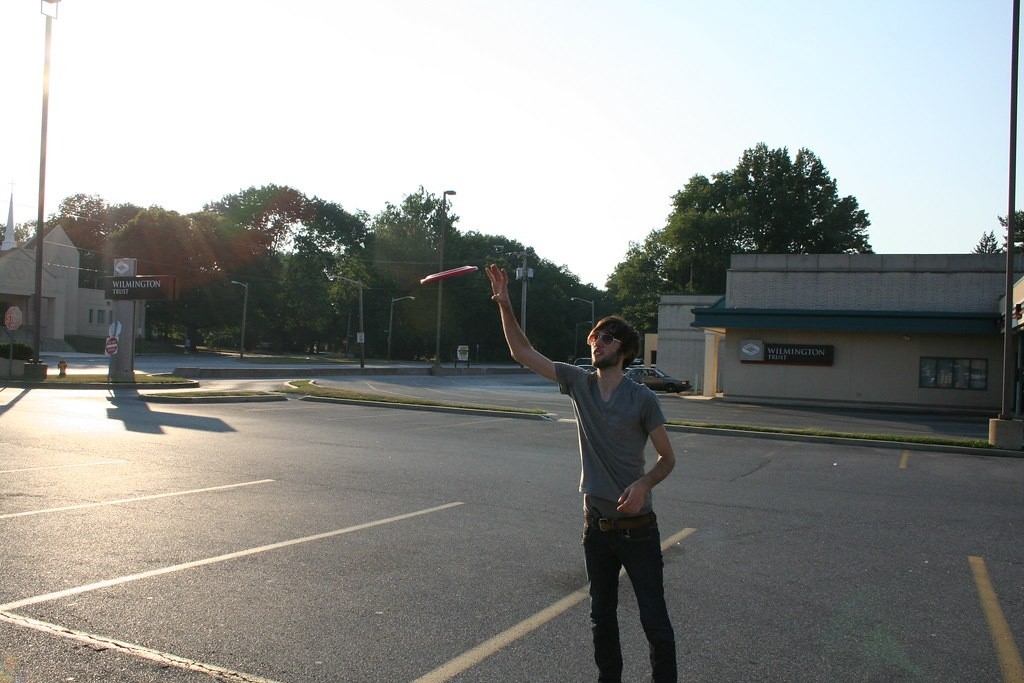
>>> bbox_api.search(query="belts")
[582,506,656,531]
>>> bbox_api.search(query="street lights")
[430,190,457,377]
[571,296,596,331]
[574,319,593,355]
[231,280,249,357]
[328,276,365,369]
[386,295,415,362]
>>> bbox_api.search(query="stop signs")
[105,337,118,354]
[5,307,23,330]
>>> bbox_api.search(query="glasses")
[589,334,621,347]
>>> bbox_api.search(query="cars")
[623,366,692,394]
[573,355,657,376]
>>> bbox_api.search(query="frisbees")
[420,266,478,285]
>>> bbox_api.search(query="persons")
[485,265,677,683]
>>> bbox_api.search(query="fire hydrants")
[57,358,67,378]
